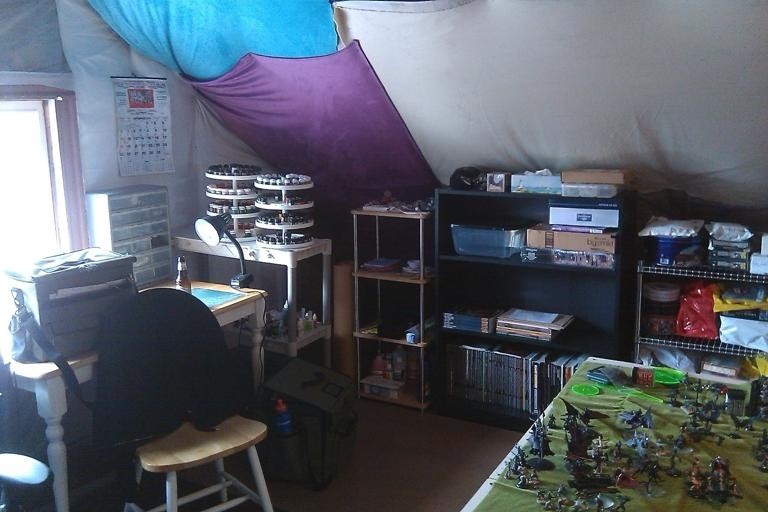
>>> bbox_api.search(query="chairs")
[97,288,274,512]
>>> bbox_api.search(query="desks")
[459,355,768,511]
[1,278,270,512]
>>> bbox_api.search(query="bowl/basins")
[407,259,419,268]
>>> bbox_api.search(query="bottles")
[175,255,191,292]
[273,399,296,435]
[642,281,682,337]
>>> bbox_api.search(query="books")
[443,302,590,415]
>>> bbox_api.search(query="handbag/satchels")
[9,287,34,362]
[261,358,359,491]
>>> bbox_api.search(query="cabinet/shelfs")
[353,187,768,429]
[86,184,173,285]
[175,231,333,371]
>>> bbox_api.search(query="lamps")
[193,213,254,290]
